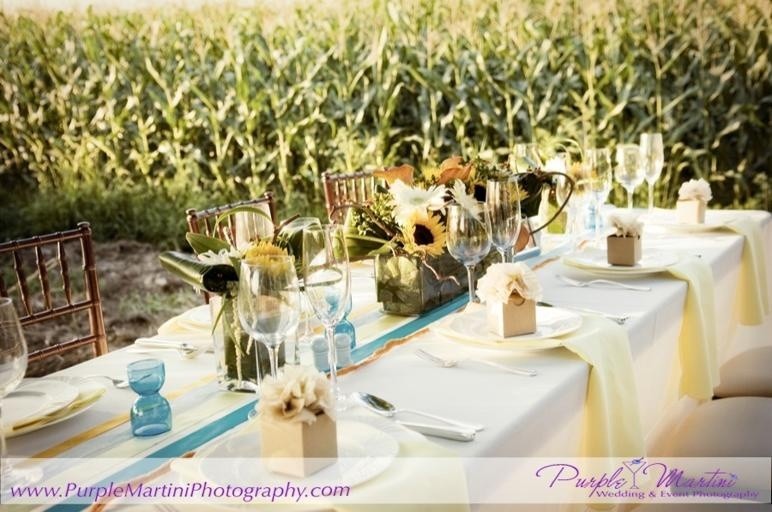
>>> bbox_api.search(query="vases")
[372,231,516,317]
[212,314,301,390]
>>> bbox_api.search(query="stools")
[713,343,771,398]
[658,394,770,506]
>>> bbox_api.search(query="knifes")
[535,300,629,324]
[135,337,215,354]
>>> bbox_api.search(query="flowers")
[335,156,525,265]
[608,209,646,240]
[677,174,716,202]
[251,360,346,429]
[476,259,544,307]
[163,209,338,314]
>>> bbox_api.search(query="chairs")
[185,192,283,305]
[321,167,383,229]
[0,219,108,379]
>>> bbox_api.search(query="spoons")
[560,274,652,291]
[360,391,483,432]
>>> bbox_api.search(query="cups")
[280,216,324,263]
[234,205,275,251]
[514,144,535,173]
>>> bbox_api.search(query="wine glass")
[486,179,522,271]
[302,223,355,415]
[444,203,492,306]
[0,298,43,496]
[552,133,665,263]
[237,255,302,420]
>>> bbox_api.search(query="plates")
[437,307,601,351]
[178,305,213,335]
[193,415,399,499]
[565,251,680,281]
[1,375,113,441]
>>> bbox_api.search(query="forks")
[416,347,538,376]
[96,372,151,388]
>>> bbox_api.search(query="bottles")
[127,359,173,436]
[332,332,353,369]
[311,335,330,372]
[326,294,356,349]
